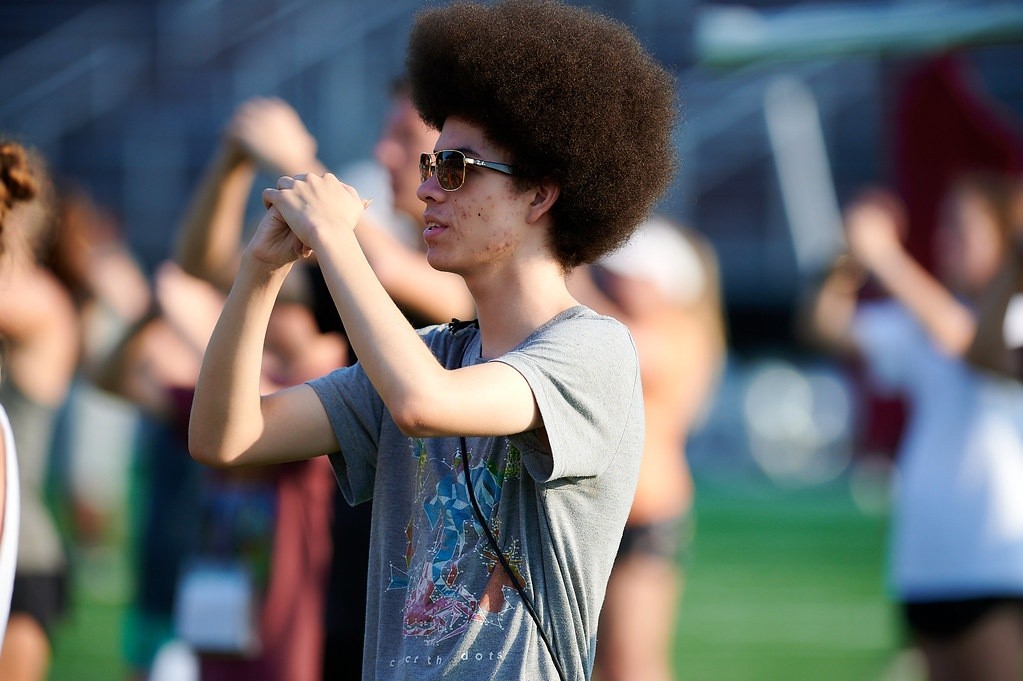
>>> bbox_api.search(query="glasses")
[419,150,512,192]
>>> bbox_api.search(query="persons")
[814,144,1023,680]
[134,69,724,681]
[1,142,151,681]
[189,2,680,681]
